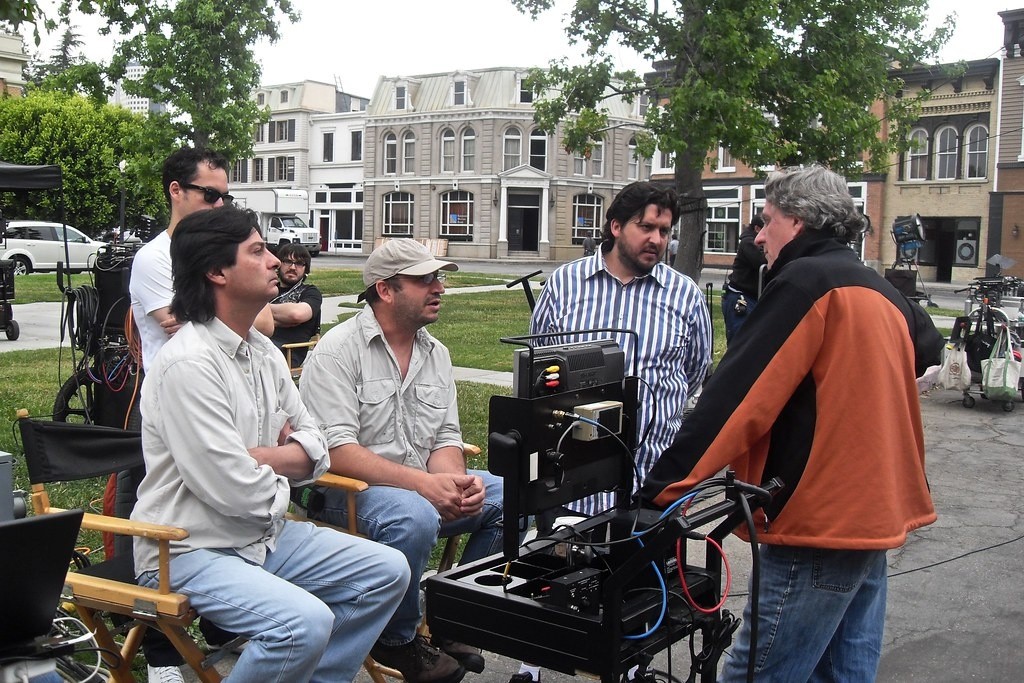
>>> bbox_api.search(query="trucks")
[228,187,310,227]
[255,212,320,258]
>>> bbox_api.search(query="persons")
[129,146,274,683]
[583,231,596,257]
[269,243,322,369]
[129,205,410,683]
[294,237,535,683]
[669,234,679,267]
[509,182,712,683]
[722,212,768,348]
[630,164,937,683]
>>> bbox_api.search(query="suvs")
[0,220,125,276]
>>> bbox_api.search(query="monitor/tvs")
[514,339,623,400]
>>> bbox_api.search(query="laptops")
[0,509,84,644]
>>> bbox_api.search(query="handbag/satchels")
[964,304,998,373]
[980,326,1022,403]
[937,342,971,391]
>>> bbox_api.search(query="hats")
[587,231,592,234]
[357,238,459,305]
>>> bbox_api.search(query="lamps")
[1012,225,1019,237]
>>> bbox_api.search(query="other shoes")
[205,634,252,654]
[147,662,184,683]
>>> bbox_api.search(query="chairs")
[14,407,482,682]
[282,329,321,379]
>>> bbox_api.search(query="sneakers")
[430,633,485,674]
[370,635,468,683]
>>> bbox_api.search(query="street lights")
[119,159,129,244]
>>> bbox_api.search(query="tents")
[0,160,71,292]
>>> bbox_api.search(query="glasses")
[391,272,447,286]
[282,259,306,268]
[183,183,234,206]
